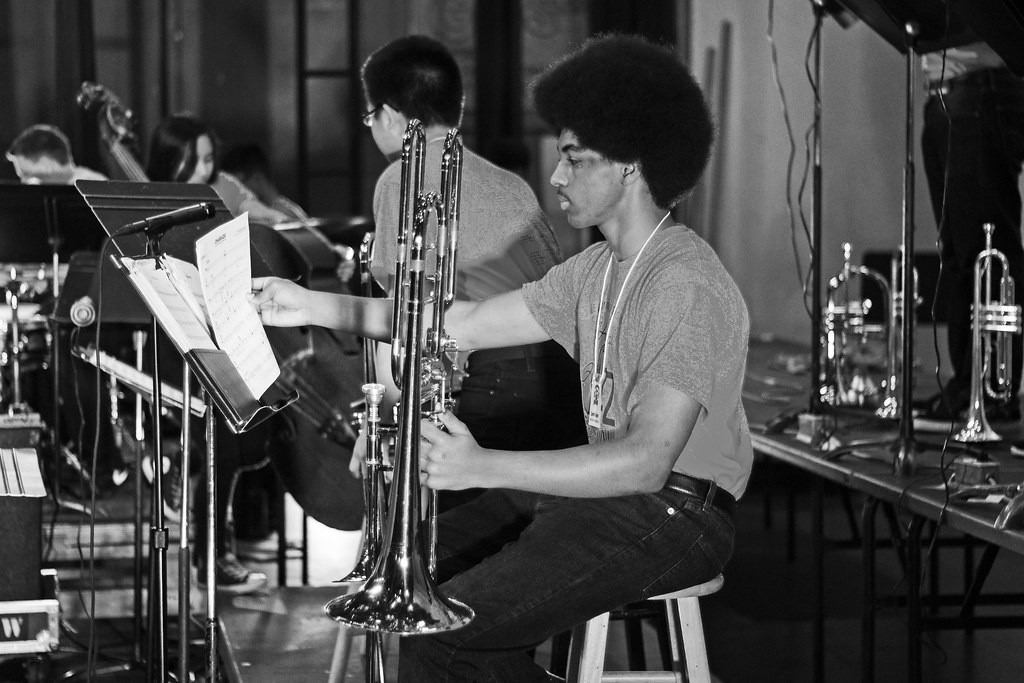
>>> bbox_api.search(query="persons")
[5,124,109,185]
[148,110,355,282]
[912,40,1023,423]
[248,30,756,683]
[361,34,572,517]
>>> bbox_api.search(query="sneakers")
[162,467,190,523]
[197,550,268,596]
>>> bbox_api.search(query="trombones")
[333,230,391,584]
[322,116,479,683]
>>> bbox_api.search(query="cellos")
[77,78,392,533]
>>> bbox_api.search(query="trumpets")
[950,223,1023,443]
[873,244,920,421]
[819,243,891,412]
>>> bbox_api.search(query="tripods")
[758,0,1005,480]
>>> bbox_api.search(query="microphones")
[116,201,216,237]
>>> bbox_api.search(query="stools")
[541,574,722,683]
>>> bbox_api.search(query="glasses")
[360,101,399,127]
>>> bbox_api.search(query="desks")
[743,334,1024,683]
